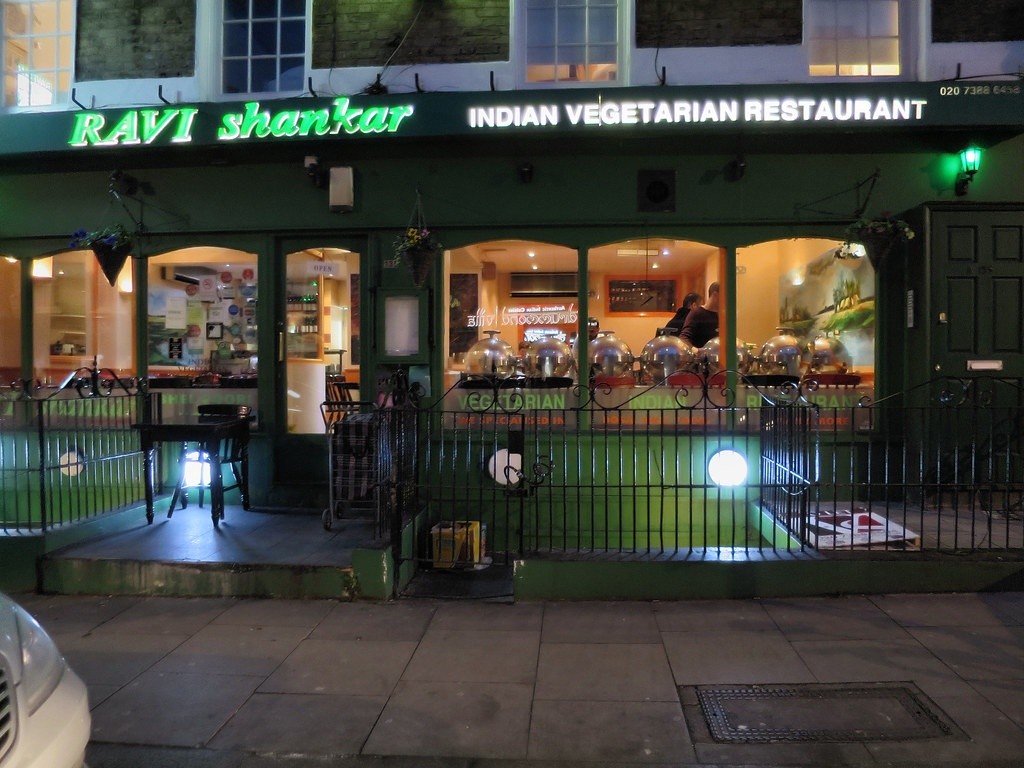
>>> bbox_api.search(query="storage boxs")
[432,521,479,569]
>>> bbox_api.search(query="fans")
[69,224,139,251]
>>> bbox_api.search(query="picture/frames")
[206,322,224,339]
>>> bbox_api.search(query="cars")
[1,593,94,768]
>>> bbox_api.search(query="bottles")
[288,316,317,334]
[288,294,316,303]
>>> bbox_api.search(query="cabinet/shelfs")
[50,314,87,358]
[286,309,318,336]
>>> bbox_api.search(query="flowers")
[392,226,445,261]
[850,207,914,242]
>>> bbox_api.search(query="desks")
[130,416,257,526]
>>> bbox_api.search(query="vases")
[89,240,132,287]
[857,233,898,275]
[398,248,435,290]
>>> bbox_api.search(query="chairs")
[741,375,801,390]
[458,377,573,389]
[668,374,726,389]
[166,404,252,520]
[590,376,637,389]
[803,373,862,389]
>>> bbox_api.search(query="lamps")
[517,146,535,183]
[105,159,127,182]
[304,144,325,186]
[729,135,747,180]
[328,166,355,214]
[707,450,753,489]
[959,139,985,196]
[107,184,143,228]
[58,447,86,477]
[486,447,522,485]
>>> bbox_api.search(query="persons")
[679,282,720,356]
[572,317,600,363]
[663,291,703,338]
